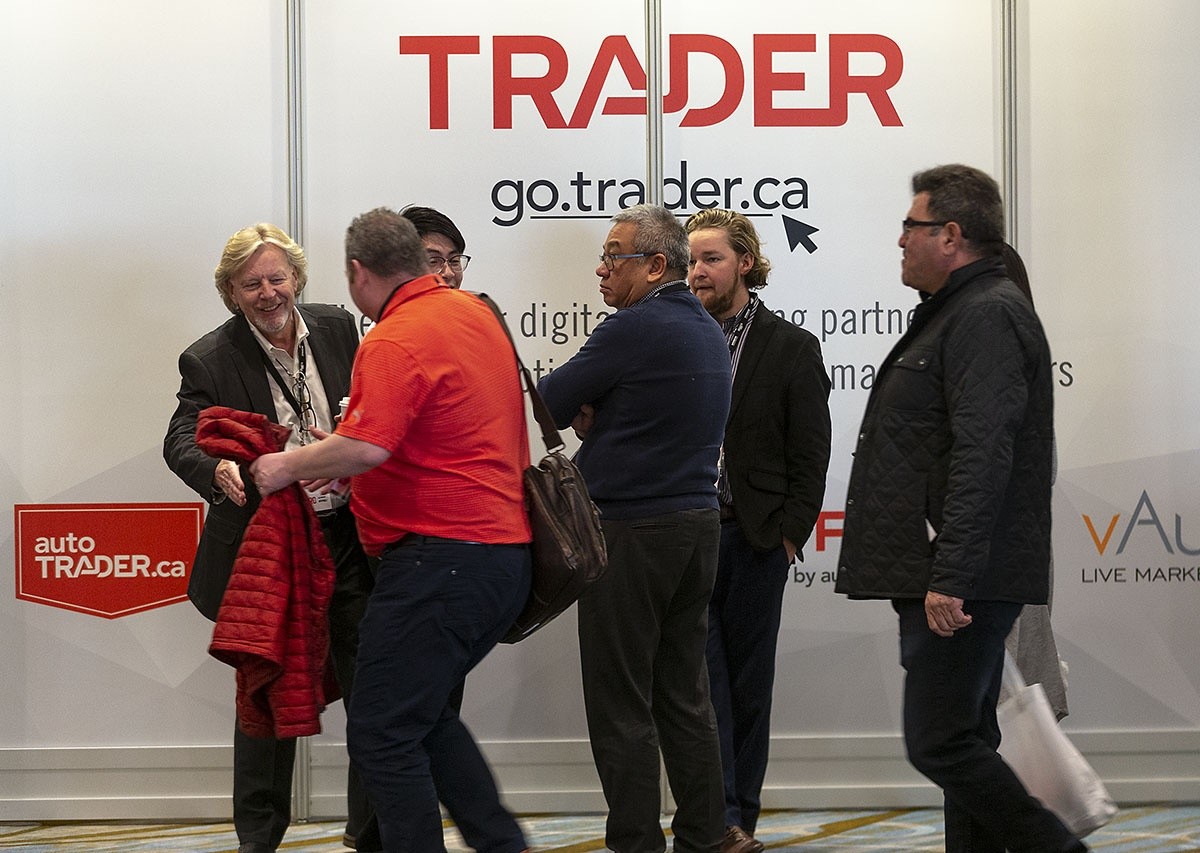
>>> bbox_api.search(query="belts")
[722,505,735,520]
[317,508,350,528]
[392,534,486,545]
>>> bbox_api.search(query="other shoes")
[722,826,765,853]
[343,832,358,848]
[238,842,275,853]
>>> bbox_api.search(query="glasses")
[600,253,657,271]
[292,382,317,431]
[903,216,970,239]
[428,254,471,274]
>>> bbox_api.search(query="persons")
[682,209,832,853]
[836,160,1091,853]
[533,205,732,853]
[250,208,532,853]
[357,206,471,852]
[163,224,374,853]
[1000,242,1067,722]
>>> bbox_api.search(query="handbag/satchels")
[498,453,608,644]
[998,650,1119,840]
